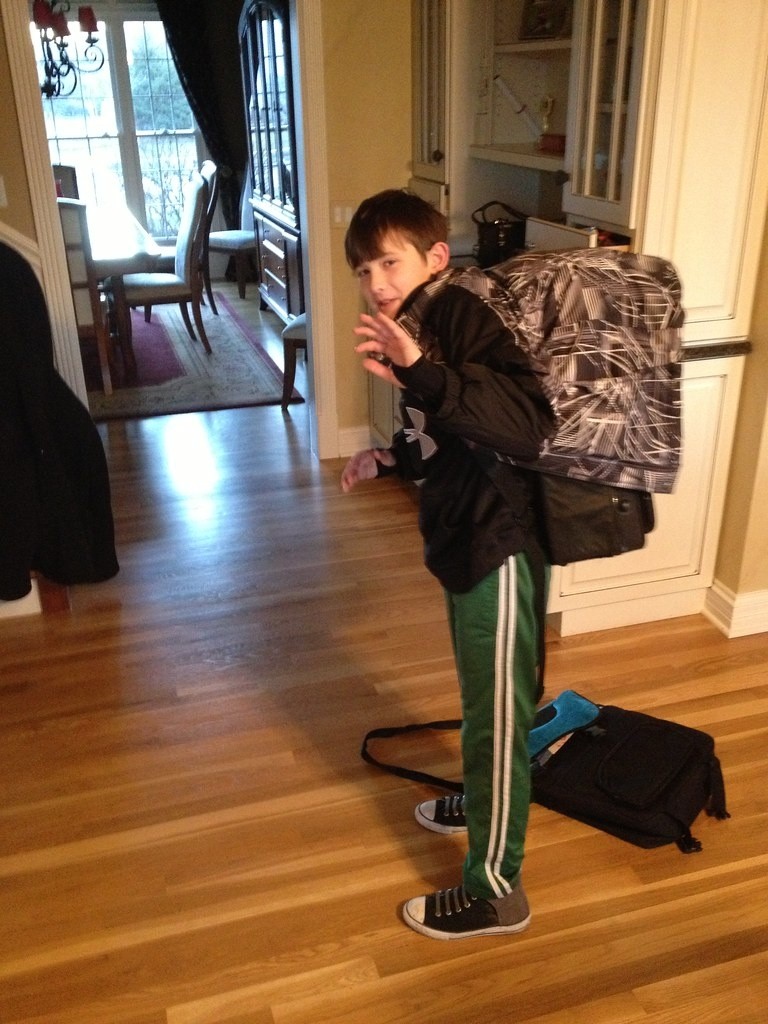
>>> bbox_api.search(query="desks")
[75,193,162,372]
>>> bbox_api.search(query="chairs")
[129,160,220,318]
[199,160,256,300]
[103,172,211,355]
[56,197,114,394]
[52,165,78,199]
[280,312,306,409]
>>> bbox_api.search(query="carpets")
[80,289,306,421]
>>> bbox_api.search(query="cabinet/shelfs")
[405,0,768,640]
[237,0,305,328]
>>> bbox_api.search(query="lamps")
[28,0,105,97]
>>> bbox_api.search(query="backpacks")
[538,477,656,568]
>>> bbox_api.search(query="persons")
[338,190,557,940]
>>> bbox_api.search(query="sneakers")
[413,794,471,836]
[402,877,531,940]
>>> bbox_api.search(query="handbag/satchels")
[526,691,727,854]
[471,201,527,266]
